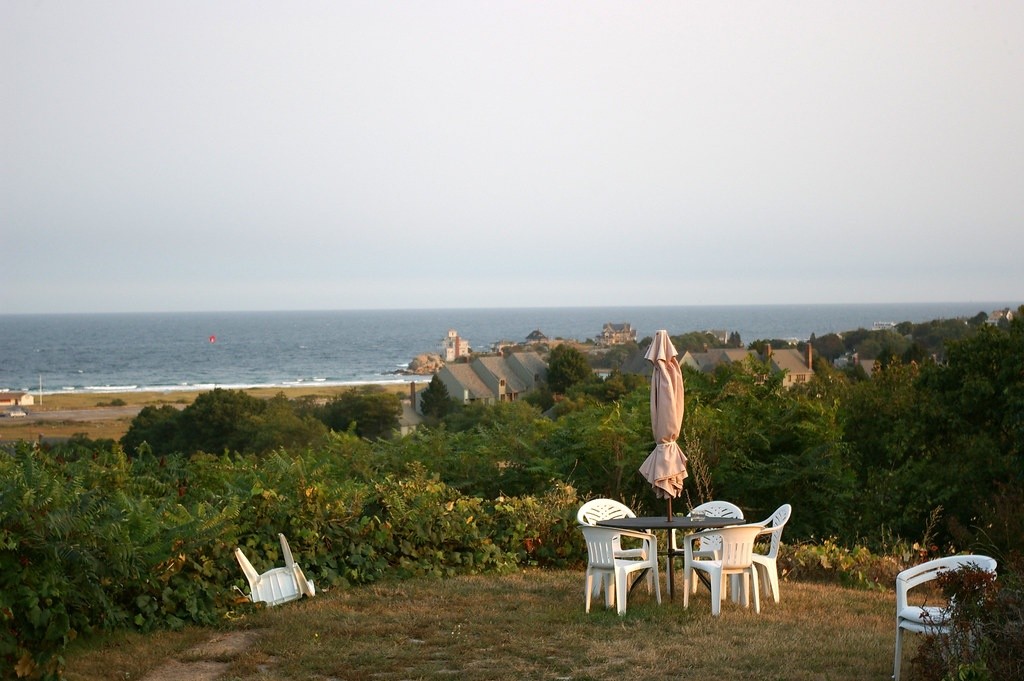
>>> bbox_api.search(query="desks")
[596,516,746,605]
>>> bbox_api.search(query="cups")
[690,511,705,522]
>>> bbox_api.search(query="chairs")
[576,525,662,616]
[891,554,997,681]
[723,503,791,605]
[577,498,653,600]
[666,500,745,596]
[232,532,316,607]
[683,525,766,616]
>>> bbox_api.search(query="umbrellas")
[639,330,689,604]
